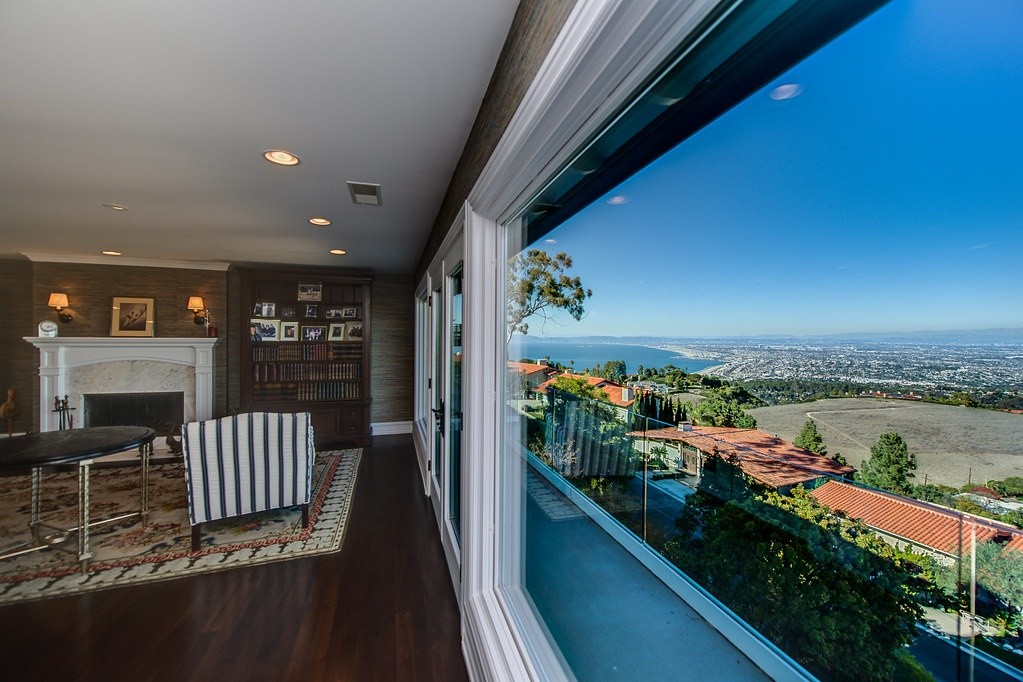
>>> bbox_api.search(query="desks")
[0,426,156,576]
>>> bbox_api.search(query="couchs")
[182,412,316,552]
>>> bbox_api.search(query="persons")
[250,300,363,342]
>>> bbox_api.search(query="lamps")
[188,296,204,325]
[48,293,73,323]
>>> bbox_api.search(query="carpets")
[0,448,364,608]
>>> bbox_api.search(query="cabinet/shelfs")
[239,273,373,446]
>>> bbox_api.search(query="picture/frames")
[108,296,156,337]
